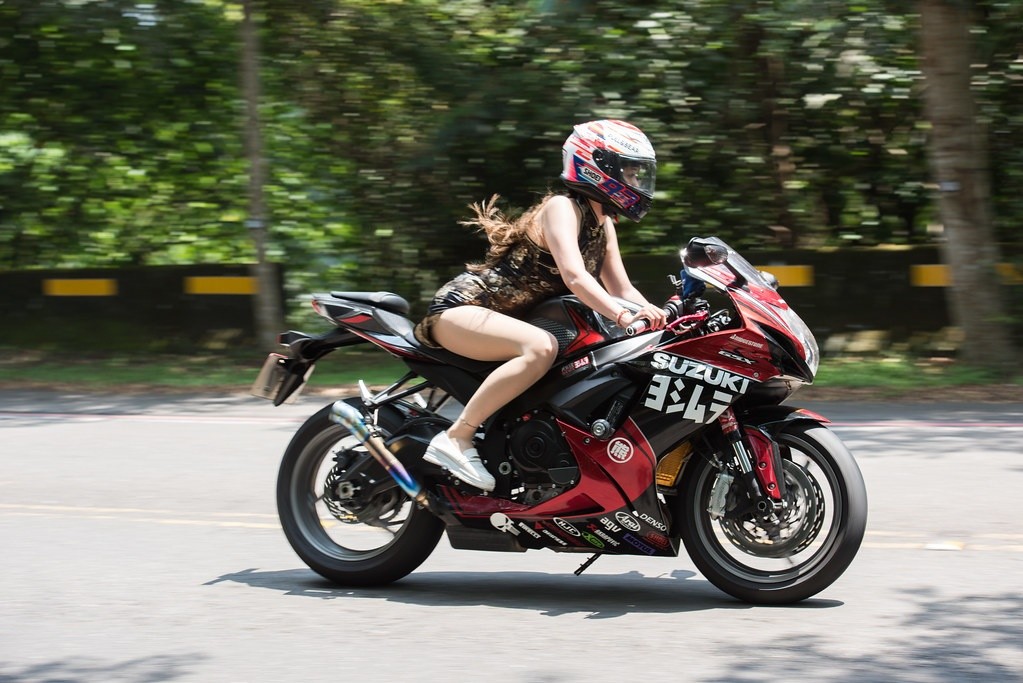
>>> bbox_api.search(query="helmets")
[558,119,658,223]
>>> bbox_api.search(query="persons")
[413,119,667,492]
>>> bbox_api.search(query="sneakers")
[421,430,496,492]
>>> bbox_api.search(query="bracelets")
[617,309,630,327]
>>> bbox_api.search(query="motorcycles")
[247,236,869,606]
